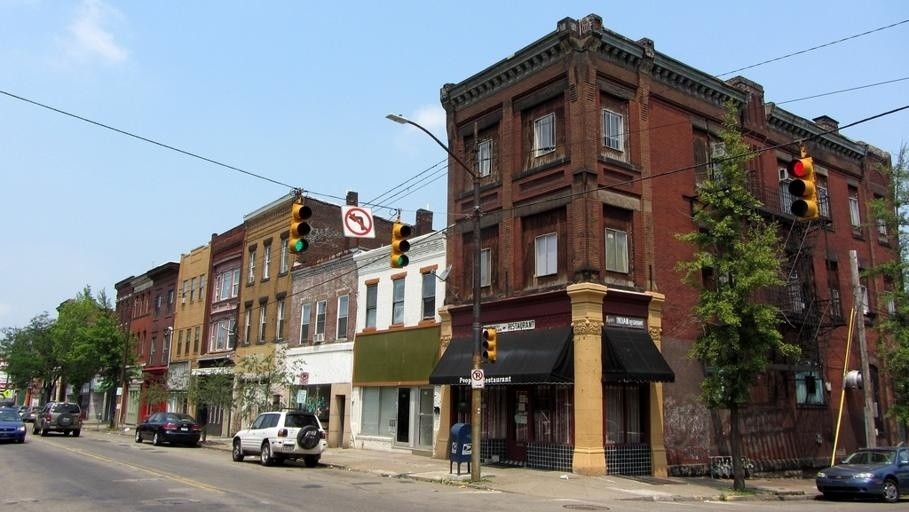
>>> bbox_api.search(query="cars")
[0,402,41,444]
[135,412,202,448]
[816,447,909,504]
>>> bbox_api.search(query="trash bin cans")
[448,423,472,476]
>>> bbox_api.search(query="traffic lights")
[390,222,410,269]
[288,202,312,254]
[482,327,496,363]
[787,156,820,221]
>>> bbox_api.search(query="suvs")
[32,401,83,437]
[232,411,328,468]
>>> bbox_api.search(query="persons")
[195,398,207,442]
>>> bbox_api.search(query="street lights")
[384,113,482,482]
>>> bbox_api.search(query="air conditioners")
[313,332,325,346]
